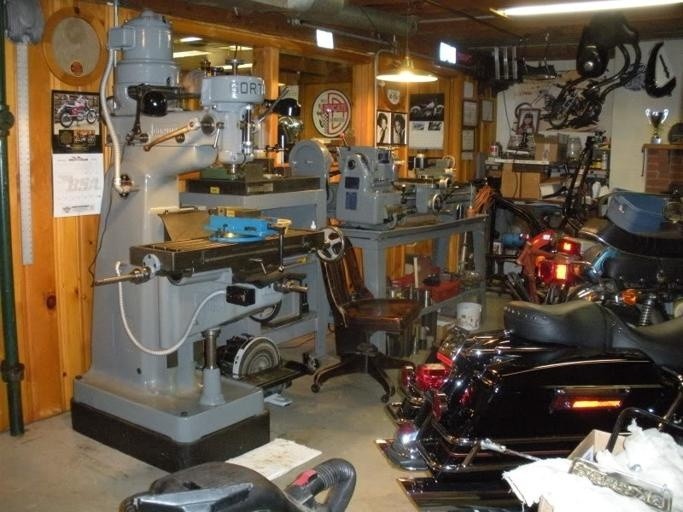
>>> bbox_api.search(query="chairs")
[312,232,424,404]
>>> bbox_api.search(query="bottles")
[565,137,608,171]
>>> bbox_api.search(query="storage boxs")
[499,163,541,201]
[419,278,459,303]
[535,142,566,163]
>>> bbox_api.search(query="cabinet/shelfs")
[495,159,608,206]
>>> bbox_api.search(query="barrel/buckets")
[456,302,482,330]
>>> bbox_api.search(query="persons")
[394,115,405,144]
[518,114,534,133]
[377,113,389,143]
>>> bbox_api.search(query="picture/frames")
[517,106,541,136]
[376,109,391,146]
[391,111,407,146]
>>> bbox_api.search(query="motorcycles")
[409,95,442,121]
[54,96,98,130]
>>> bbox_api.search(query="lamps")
[375,0,437,82]
